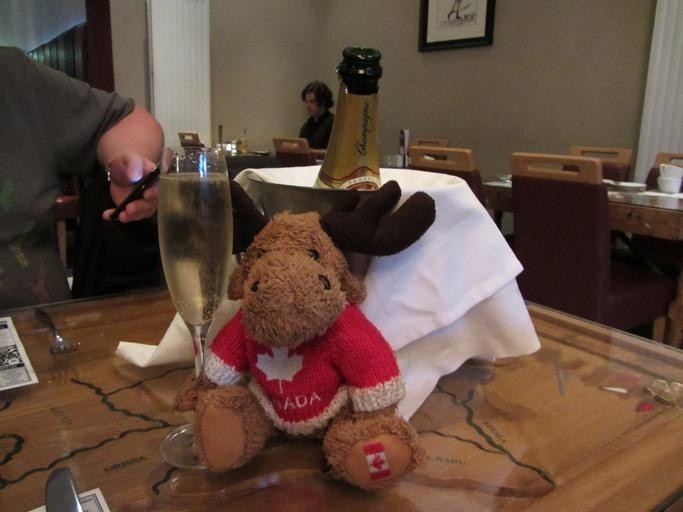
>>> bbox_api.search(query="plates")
[602,178,648,193]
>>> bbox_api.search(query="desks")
[1,283,683,512]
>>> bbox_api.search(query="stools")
[54,193,81,270]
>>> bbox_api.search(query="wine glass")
[156,146,233,471]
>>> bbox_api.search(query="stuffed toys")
[168,180,436,494]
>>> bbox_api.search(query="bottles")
[313,46,383,192]
[241,128,249,153]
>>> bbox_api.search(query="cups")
[658,163,683,178]
[657,176,682,193]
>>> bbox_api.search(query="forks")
[33,307,80,355]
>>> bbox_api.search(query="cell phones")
[109,161,161,220]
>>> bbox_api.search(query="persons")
[0,33,170,313]
[296,78,336,147]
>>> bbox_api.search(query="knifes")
[44,466,82,512]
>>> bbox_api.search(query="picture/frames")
[416,0,498,54]
